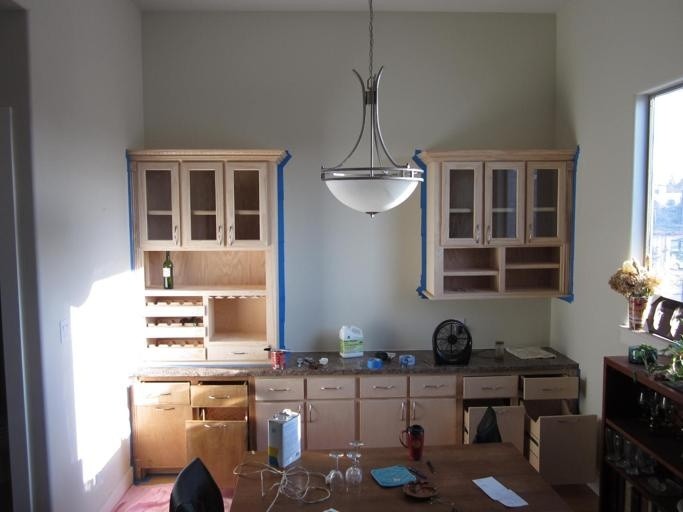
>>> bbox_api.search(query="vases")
[628,295,647,329]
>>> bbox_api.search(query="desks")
[229,441,579,511]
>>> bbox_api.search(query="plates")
[370,465,416,489]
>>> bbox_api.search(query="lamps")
[319,1,426,218]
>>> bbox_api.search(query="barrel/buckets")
[338,325,364,358]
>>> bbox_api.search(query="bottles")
[161,252,173,289]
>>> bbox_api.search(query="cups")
[399,426,424,461]
[606,429,655,475]
[496,341,504,361]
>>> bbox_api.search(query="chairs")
[170,457,224,512]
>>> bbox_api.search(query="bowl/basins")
[367,358,383,369]
[630,345,657,365]
[401,480,440,499]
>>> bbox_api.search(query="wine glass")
[325,450,344,492]
[349,441,365,452]
[639,390,674,433]
[345,450,362,485]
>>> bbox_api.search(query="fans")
[432,319,473,366]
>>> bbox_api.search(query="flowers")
[609,268,653,300]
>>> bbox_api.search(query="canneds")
[271,349,286,371]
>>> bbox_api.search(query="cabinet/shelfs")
[412,147,579,304]
[127,375,254,482]
[126,148,292,367]
[254,376,359,451]
[359,375,458,449]
[597,355,682,510]
[462,376,598,486]
